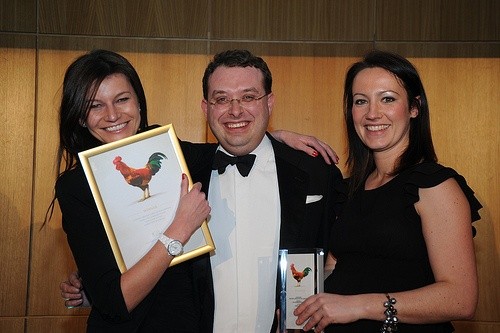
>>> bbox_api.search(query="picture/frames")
[78,123,216,275]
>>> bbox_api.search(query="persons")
[275,49,483,333]
[59,50,344,333]
[39,50,339,333]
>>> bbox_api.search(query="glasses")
[207,92,270,110]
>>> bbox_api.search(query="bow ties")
[211,149,257,177]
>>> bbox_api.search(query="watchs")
[158,234,182,257]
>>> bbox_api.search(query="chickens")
[113,152,168,201]
[290,263,313,287]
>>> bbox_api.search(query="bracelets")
[380,293,399,333]
